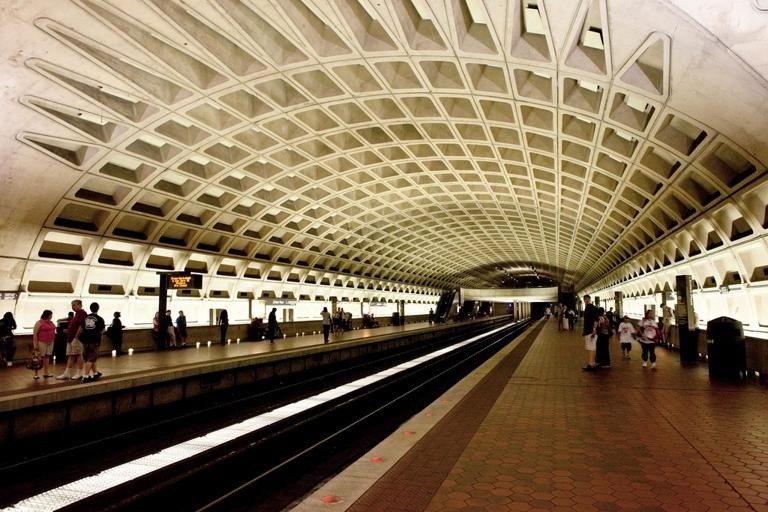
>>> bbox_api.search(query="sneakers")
[56,373,102,383]
[623,351,631,359]
[32,373,54,380]
[581,360,612,371]
[640,358,658,370]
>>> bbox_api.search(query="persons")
[0,312,17,368]
[176,310,188,346]
[111,311,126,356]
[72,301,105,383]
[32,310,54,379]
[54,299,85,380]
[269,307,277,344]
[166,310,177,349]
[153,312,159,331]
[320,307,380,343]
[218,309,229,346]
[543,294,674,374]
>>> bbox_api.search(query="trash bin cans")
[706,316,747,383]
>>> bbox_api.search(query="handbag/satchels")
[25,348,45,371]
